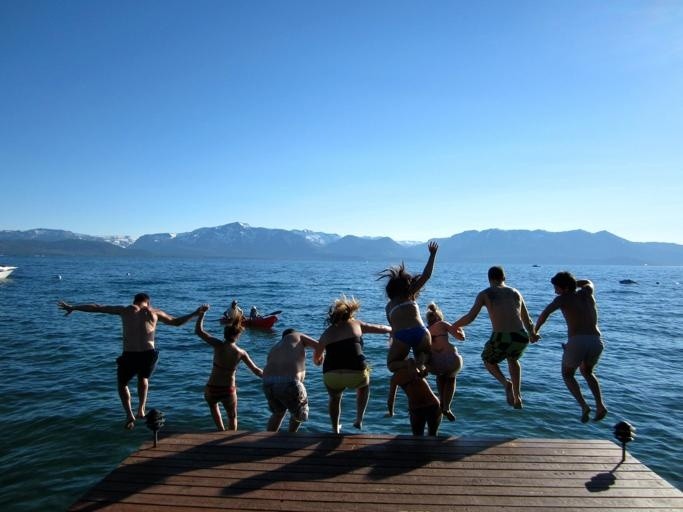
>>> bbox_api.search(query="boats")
[0,264,17,280]
[220,313,278,331]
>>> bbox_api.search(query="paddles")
[262,311,282,316]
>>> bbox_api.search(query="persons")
[259,327,324,432]
[422,302,465,423]
[195,302,263,431]
[529,270,608,422]
[383,240,439,382]
[221,298,243,320]
[311,293,392,435]
[450,265,541,410]
[56,292,202,431]
[248,304,259,320]
[385,355,443,438]
